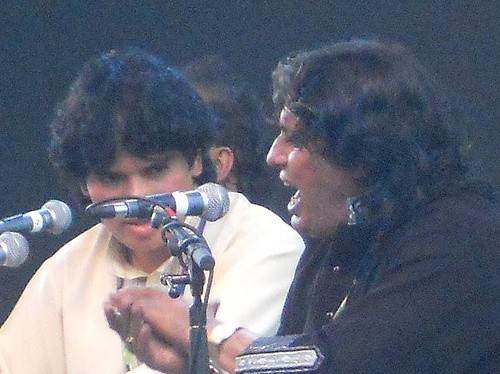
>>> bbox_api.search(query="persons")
[175,51,264,209]
[106,37,500,373]
[1,46,306,374]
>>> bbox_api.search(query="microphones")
[0,197,72,235]
[84,182,230,223]
[0,232,30,268]
